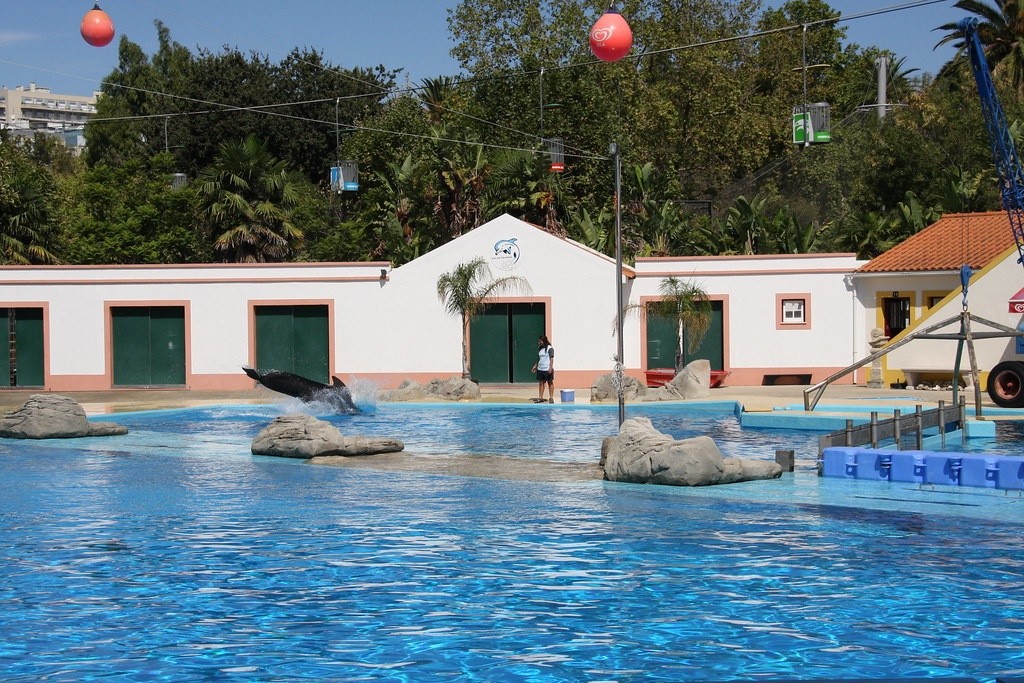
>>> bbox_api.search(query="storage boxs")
[560,389,575,402]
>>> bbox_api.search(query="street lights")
[609,142,624,432]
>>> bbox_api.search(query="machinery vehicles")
[955,16,1024,407]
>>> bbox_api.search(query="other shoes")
[535,399,544,403]
[549,398,554,403]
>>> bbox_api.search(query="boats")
[644,369,732,388]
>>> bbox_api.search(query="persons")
[532,335,554,404]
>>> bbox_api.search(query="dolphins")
[241,364,360,412]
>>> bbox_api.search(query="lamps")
[381,269,388,277]
